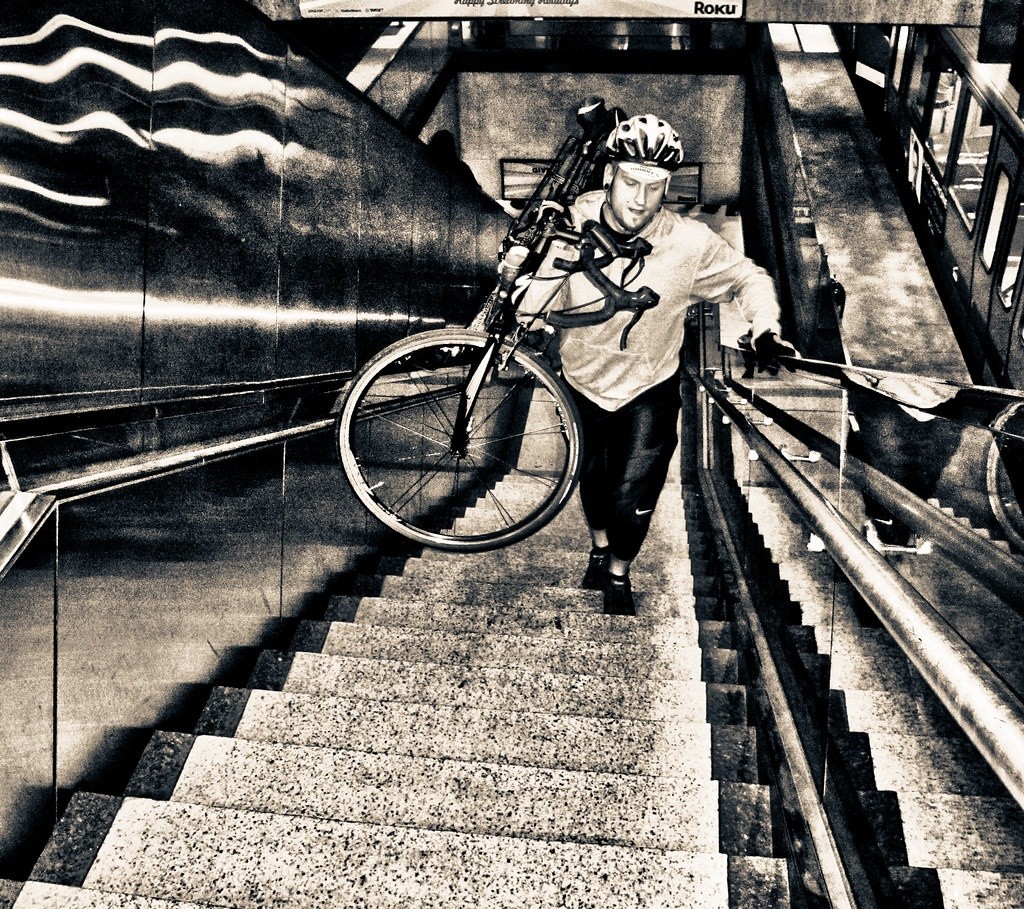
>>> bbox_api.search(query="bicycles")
[329,97,665,551]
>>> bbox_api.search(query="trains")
[883,22,1023,391]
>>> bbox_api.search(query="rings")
[541,201,548,206]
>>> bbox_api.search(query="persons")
[511,114,802,617]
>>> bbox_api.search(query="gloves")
[755,331,801,374]
[536,199,575,234]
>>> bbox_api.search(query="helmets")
[603,113,685,172]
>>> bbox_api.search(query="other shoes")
[603,572,636,616]
[582,549,610,590]
